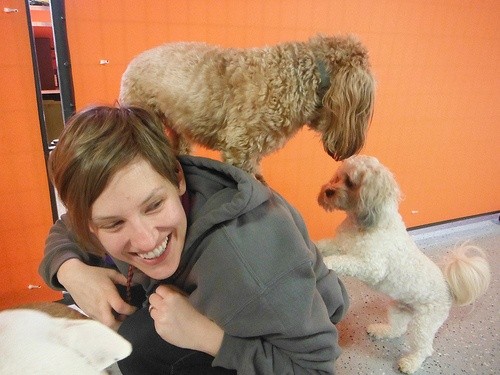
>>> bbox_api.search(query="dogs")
[314,154,491,374]
[120,35,375,178]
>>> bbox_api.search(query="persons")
[35,106,350,375]
